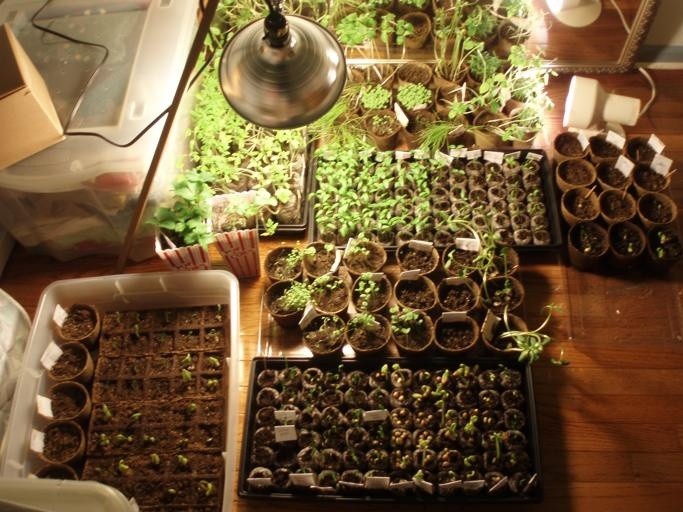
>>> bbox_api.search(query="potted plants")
[551,129,681,268]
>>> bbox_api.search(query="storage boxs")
[1,268,240,512]
[0,22,67,172]
[0,0,203,264]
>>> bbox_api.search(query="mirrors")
[210,1,660,77]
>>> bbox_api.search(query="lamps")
[216,0,348,132]
[546,0,603,29]
[563,73,642,138]
